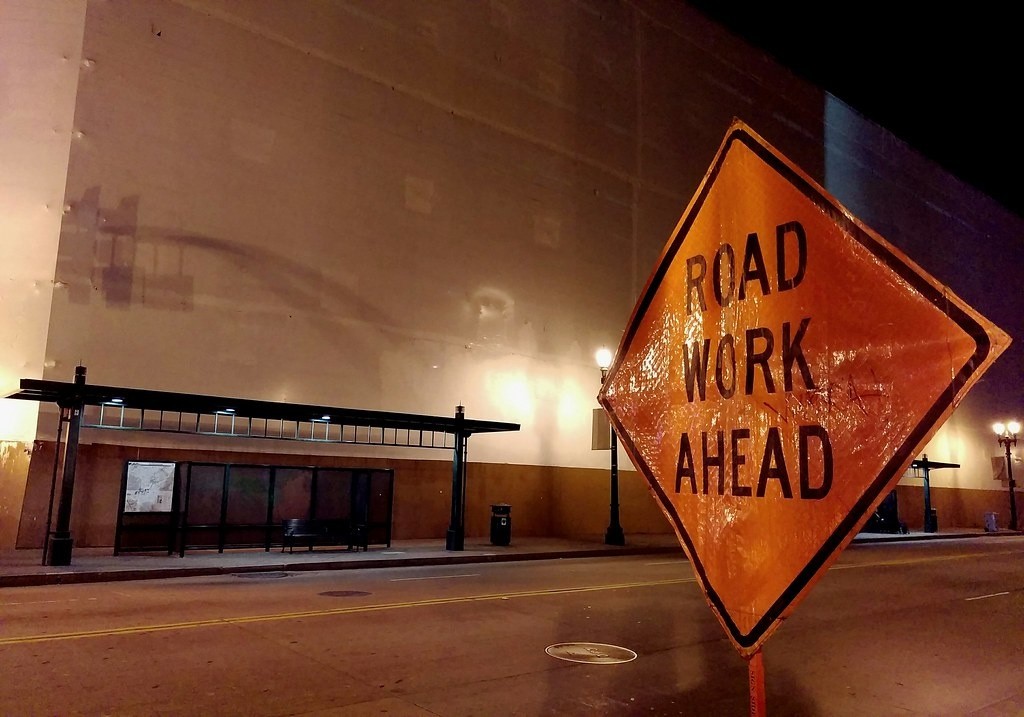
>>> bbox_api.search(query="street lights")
[992,421,1022,531]
[594,348,625,546]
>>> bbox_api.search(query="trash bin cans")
[490,502,513,546]
[931,507,937,532]
